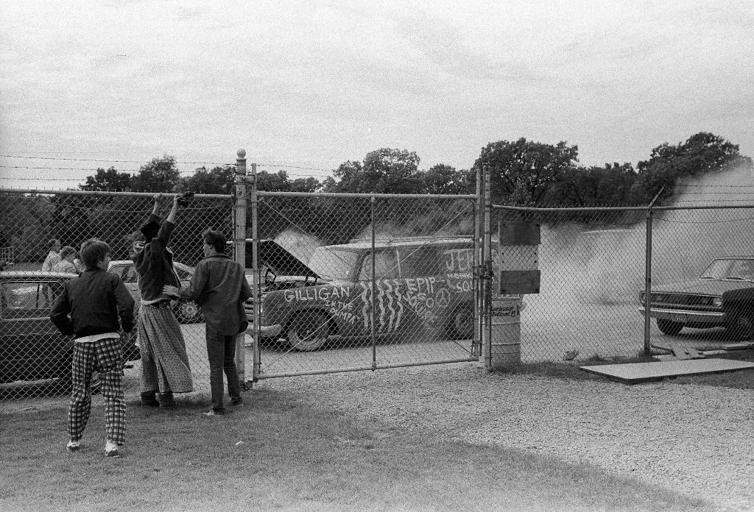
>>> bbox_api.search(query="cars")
[637,254,754,339]
[106,260,208,324]
[0,270,141,397]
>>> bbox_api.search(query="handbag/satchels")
[169,271,182,300]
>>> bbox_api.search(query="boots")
[141,393,176,409]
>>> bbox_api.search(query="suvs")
[224,239,501,351]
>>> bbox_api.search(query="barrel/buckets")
[479,294,521,369]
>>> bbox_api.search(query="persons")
[162,227,253,417]
[42,238,85,274]
[49,238,135,457]
[128,192,194,411]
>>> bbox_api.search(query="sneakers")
[105,442,118,457]
[203,409,225,417]
[227,397,243,406]
[67,440,80,448]
